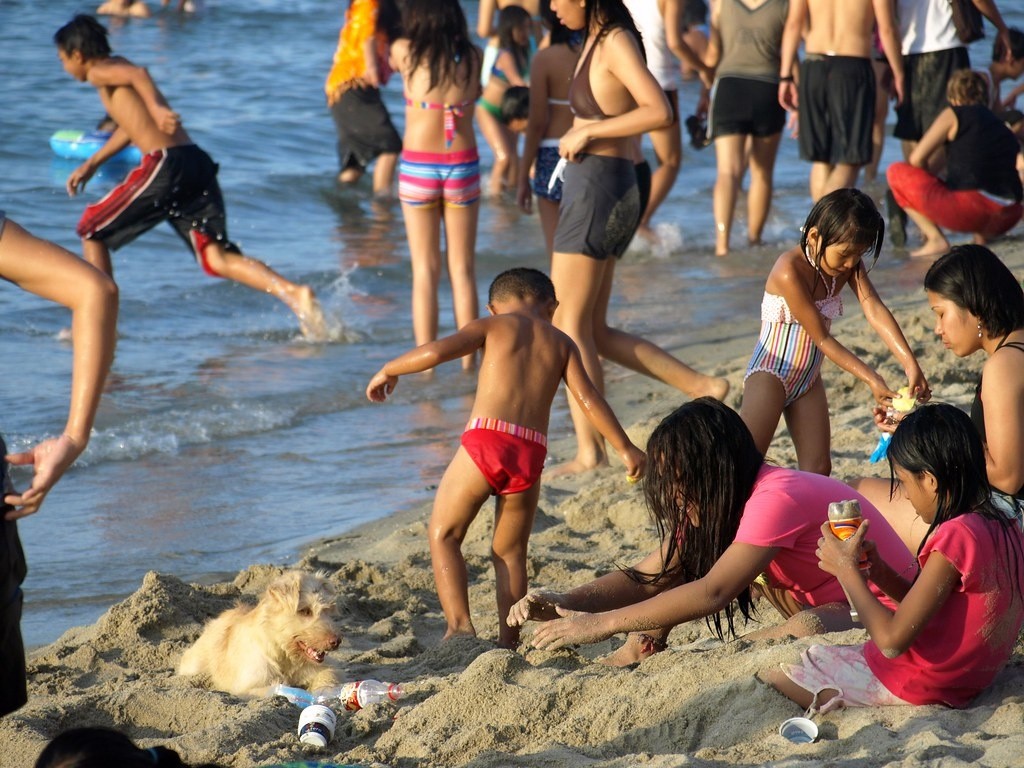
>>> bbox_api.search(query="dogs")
[175,568,345,695]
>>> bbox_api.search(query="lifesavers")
[48,129,144,164]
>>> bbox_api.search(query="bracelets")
[777,75,795,82]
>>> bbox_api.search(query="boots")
[886,189,907,246]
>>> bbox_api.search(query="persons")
[389,1,485,374]
[679,1,710,81]
[504,395,919,665]
[0,212,119,721]
[739,188,931,476]
[550,0,730,470]
[326,0,394,203]
[756,403,1024,719]
[778,0,908,210]
[54,15,326,389]
[473,6,535,211]
[517,0,586,271]
[894,0,1012,244]
[846,243,1024,561]
[986,26,1024,160]
[367,269,648,661]
[700,0,800,260]
[476,0,543,93]
[885,71,1024,258]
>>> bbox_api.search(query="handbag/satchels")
[951,0,986,42]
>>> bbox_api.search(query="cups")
[779,717,819,744]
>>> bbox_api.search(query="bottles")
[311,679,403,711]
[829,500,872,622]
[299,705,337,747]
